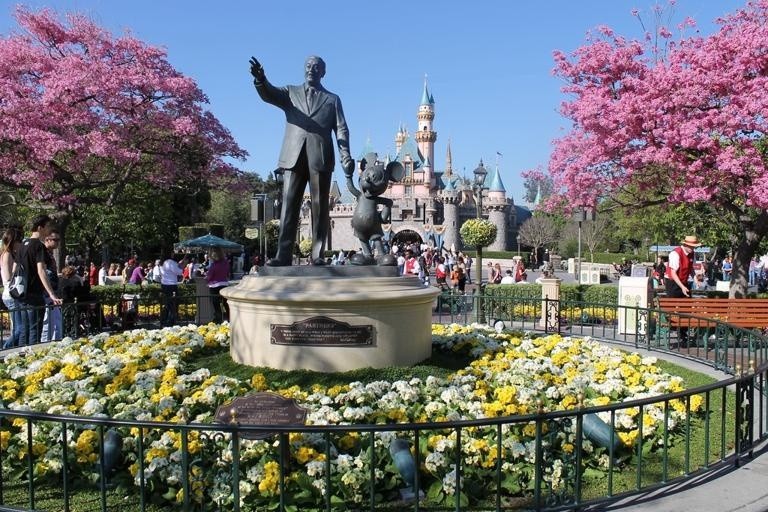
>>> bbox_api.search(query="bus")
[647,245,712,270]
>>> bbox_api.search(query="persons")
[691,252,768,298]
[249,55,356,267]
[199,247,273,324]
[619,260,633,277]
[664,235,702,348]
[487,248,550,286]
[611,257,628,276]
[331,239,473,293]
[653,255,666,290]
[98,252,197,329]
[1,214,104,351]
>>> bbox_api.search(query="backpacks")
[9,245,28,300]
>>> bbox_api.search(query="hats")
[681,235,702,247]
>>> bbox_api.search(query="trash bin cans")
[581,265,601,285]
[568,258,579,274]
[617,276,653,336]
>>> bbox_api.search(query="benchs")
[653,298,768,348]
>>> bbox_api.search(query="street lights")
[516,234,521,256]
[573,205,585,283]
[472,157,489,324]
